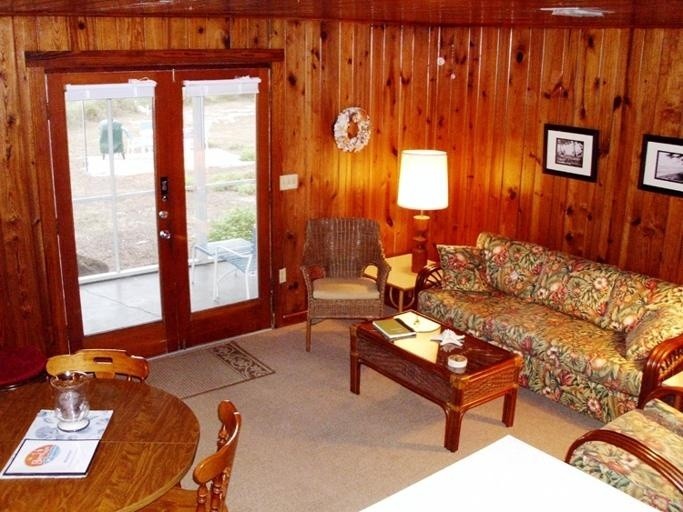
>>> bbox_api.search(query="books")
[372,317,416,339]
[0,409,114,479]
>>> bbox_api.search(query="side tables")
[661,371,682,412]
[363,253,444,314]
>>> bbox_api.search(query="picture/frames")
[542,124,599,182]
[637,134,683,197]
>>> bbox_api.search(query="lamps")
[395,149,449,273]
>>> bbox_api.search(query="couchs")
[413,232,683,424]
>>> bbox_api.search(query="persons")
[556,139,583,158]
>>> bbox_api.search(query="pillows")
[624,304,683,360]
[435,244,493,293]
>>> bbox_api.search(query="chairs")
[97,119,129,159]
[44,348,150,385]
[299,217,392,352]
[563,385,683,512]
[133,400,242,512]
[191,223,258,302]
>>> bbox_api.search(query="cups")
[49,366,94,435]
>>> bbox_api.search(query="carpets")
[77,340,276,400]
